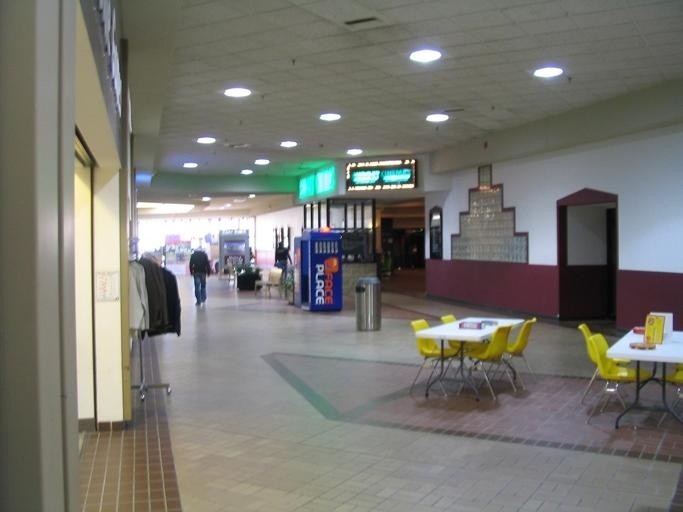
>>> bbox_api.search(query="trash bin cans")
[356,277,381,332]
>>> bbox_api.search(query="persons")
[188,244,211,306]
[274,241,292,279]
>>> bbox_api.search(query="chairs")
[253,267,286,300]
[410,310,682,429]
